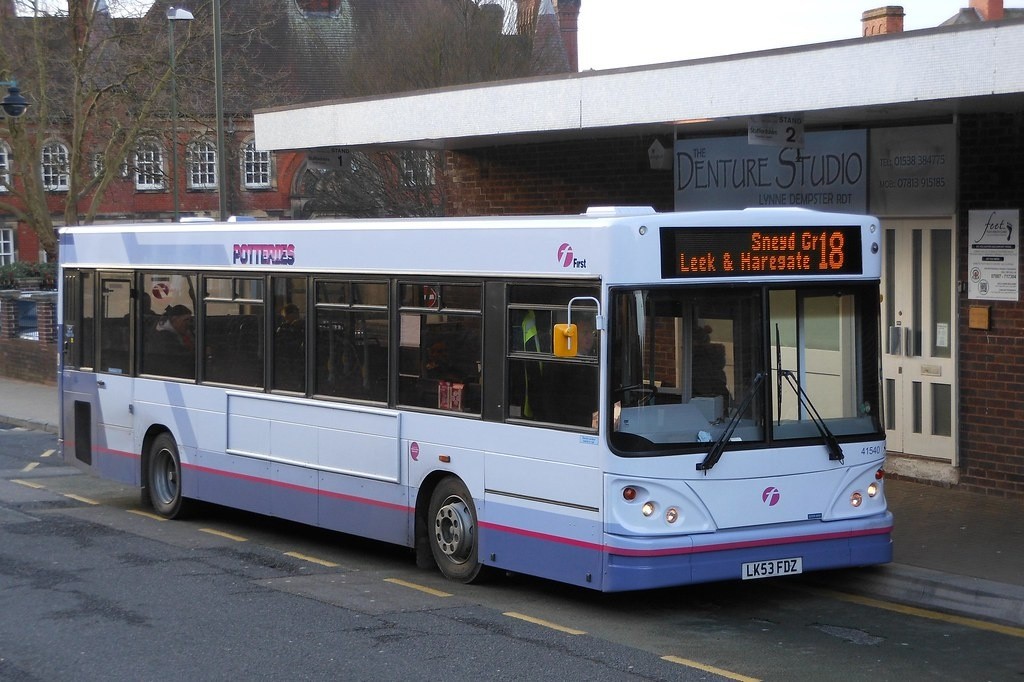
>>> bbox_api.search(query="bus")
[57,204,896,594]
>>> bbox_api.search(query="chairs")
[84,313,480,414]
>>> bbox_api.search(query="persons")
[277,303,306,390]
[545,318,599,425]
[123,291,216,381]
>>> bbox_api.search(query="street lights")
[166,6,195,220]
